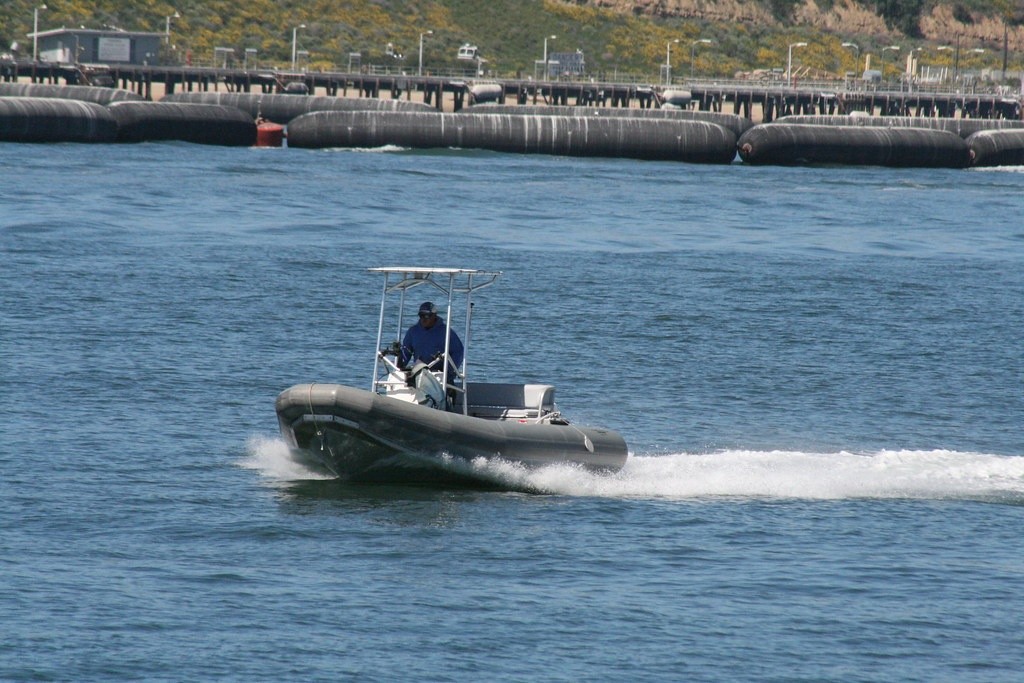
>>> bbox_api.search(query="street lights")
[937,45,954,94]
[963,48,985,95]
[666,38,681,85]
[842,43,859,91]
[880,46,901,83]
[290,23,307,72]
[32,3,47,63]
[164,13,179,46]
[544,34,556,83]
[909,47,922,93]
[691,39,710,80]
[418,31,433,76]
[787,42,808,88]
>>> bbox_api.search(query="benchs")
[453,382,555,424]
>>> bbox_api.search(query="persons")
[397,302,464,411]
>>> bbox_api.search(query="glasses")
[419,313,432,319]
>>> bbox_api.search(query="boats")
[274,266,629,488]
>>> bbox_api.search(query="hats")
[418,302,437,314]
